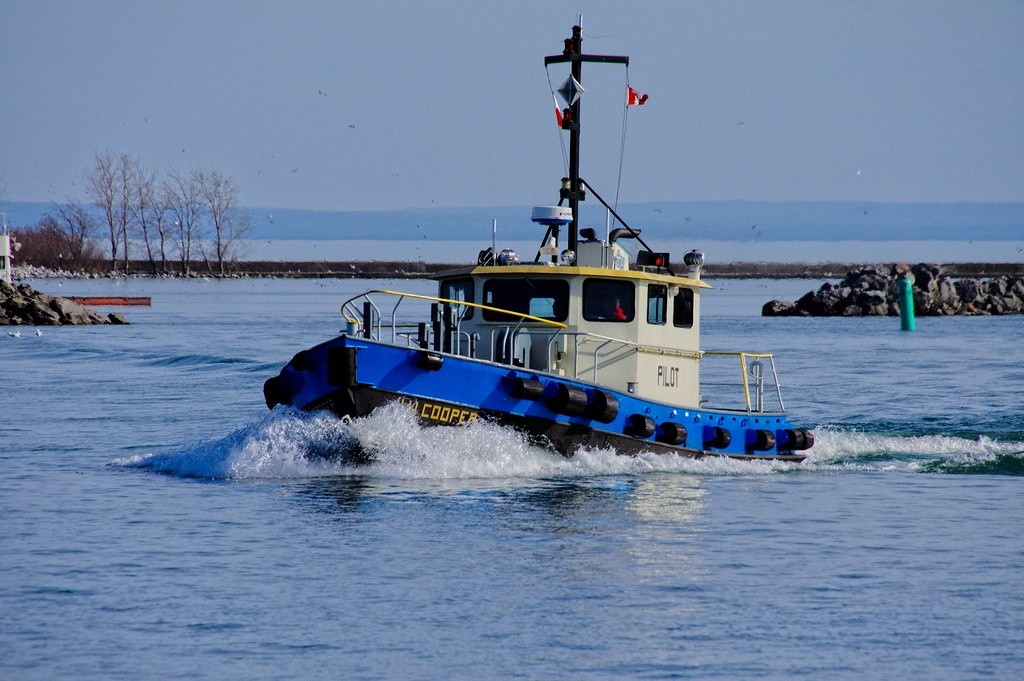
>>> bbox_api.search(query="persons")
[607,297,627,320]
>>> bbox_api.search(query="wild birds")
[7,90,438,338]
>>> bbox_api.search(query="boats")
[263,14,816,465]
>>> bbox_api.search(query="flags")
[625,84,649,108]
[551,92,564,127]
